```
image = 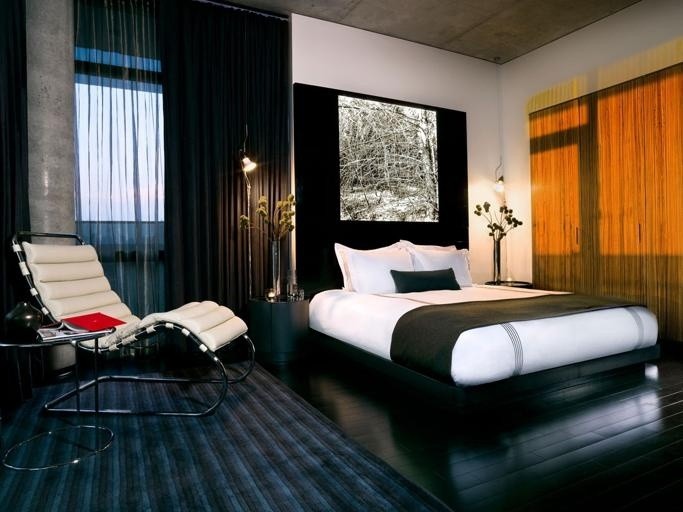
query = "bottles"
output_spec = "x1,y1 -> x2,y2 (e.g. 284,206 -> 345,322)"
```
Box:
5,301 -> 45,345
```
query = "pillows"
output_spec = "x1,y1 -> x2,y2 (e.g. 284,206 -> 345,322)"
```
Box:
335,239 -> 474,293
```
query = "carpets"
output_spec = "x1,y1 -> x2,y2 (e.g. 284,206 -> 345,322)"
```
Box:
0,360 -> 453,512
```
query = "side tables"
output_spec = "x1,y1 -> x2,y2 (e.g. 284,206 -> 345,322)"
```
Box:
0,324 -> 115,471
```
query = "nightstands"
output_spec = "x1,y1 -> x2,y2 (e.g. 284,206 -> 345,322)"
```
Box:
485,280 -> 532,290
254,299 -> 309,363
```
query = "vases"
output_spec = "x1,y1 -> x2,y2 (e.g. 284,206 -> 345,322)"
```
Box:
494,240 -> 501,284
272,240 -> 281,302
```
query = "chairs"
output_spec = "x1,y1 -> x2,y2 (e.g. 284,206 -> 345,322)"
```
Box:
13,230 -> 256,417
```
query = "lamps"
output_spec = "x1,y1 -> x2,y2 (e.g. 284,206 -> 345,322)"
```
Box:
241,155 -> 258,300
496,176 -> 515,282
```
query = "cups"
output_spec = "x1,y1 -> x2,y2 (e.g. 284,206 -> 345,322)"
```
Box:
264,289 -> 277,303
286,283 -> 297,297
294,289 -> 305,301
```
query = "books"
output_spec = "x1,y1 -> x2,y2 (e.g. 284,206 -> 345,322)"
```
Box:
41,312 -> 127,333
35,328 -> 115,342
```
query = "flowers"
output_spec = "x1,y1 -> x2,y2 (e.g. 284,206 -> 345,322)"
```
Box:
239,194 -> 296,281
474,202 -> 523,278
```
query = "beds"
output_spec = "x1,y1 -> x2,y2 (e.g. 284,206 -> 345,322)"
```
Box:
309,284 -> 662,388
